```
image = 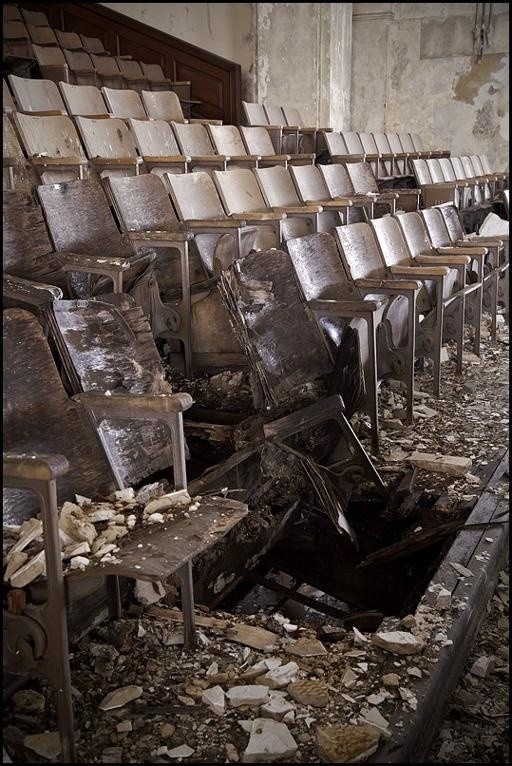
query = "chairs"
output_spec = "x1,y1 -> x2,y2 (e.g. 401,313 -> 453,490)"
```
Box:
1,111 -> 510,500
2,6 -> 24,23
242,101 -> 305,128
21,8 -> 50,26
2,74 -> 223,124
2,23 -> 131,61
1,308 -> 250,765
3,43 -> 192,116
